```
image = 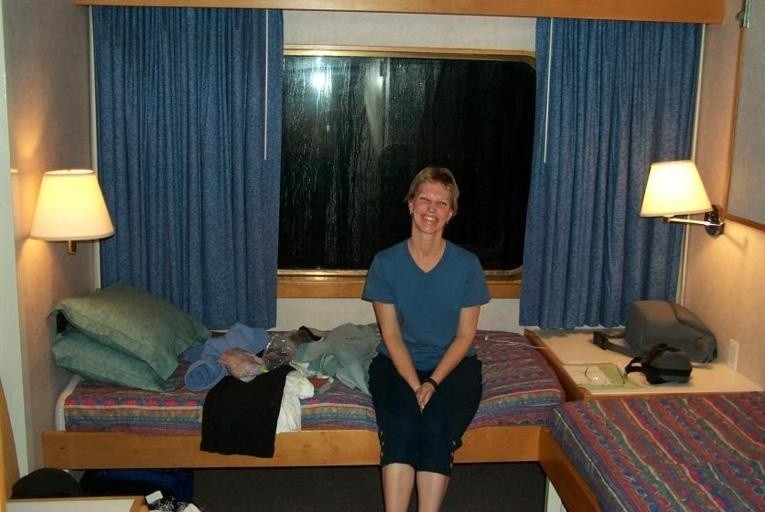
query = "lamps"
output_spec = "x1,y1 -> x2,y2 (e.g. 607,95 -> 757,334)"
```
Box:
20,167 -> 118,258
637,156 -> 727,238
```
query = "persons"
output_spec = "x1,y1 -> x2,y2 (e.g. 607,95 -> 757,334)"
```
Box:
289,95 -> 365,268
362,166 -> 491,511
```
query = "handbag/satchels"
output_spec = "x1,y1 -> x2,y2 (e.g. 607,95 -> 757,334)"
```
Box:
624,344 -> 692,385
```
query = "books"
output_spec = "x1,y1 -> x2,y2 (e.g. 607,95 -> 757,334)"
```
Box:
562,361 -> 643,395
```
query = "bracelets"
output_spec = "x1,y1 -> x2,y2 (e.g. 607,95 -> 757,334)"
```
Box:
423,377 -> 439,391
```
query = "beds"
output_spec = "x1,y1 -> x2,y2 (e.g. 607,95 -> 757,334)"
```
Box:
42,327 -> 566,475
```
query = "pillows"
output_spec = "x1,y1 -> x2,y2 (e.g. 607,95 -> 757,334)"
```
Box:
48,278 -> 214,398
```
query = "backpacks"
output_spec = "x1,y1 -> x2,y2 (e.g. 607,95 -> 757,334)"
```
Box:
592,300 -> 717,363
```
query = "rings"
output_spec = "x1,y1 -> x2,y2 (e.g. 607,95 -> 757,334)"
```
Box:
419,400 -> 424,405
